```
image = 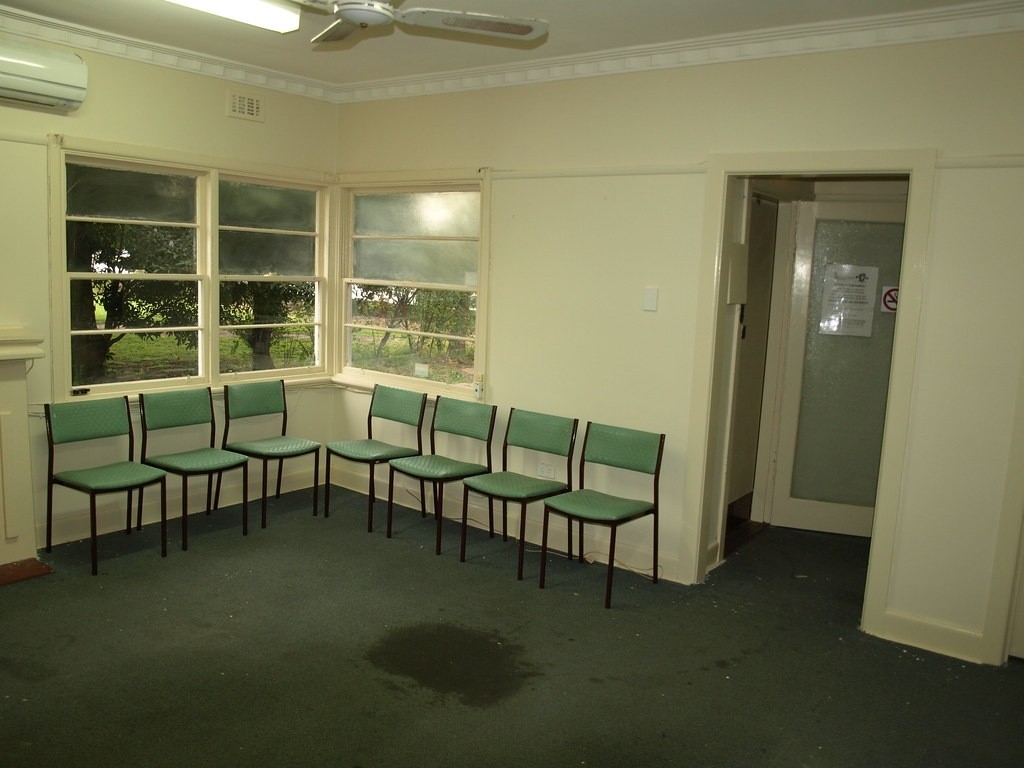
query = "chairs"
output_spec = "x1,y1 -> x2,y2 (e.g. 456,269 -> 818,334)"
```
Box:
213,378 -> 322,530
537,419 -> 668,609
386,394 -> 498,556
459,406 -> 581,581
324,382 -> 428,534
136,385 -> 251,553
40,392 -> 169,576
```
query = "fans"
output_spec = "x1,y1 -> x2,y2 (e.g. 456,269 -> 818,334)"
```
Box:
264,0 -> 552,47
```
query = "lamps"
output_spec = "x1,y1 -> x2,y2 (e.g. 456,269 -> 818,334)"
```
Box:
163,0 -> 302,35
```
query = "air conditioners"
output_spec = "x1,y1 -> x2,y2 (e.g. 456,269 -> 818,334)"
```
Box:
0,42 -> 90,116
